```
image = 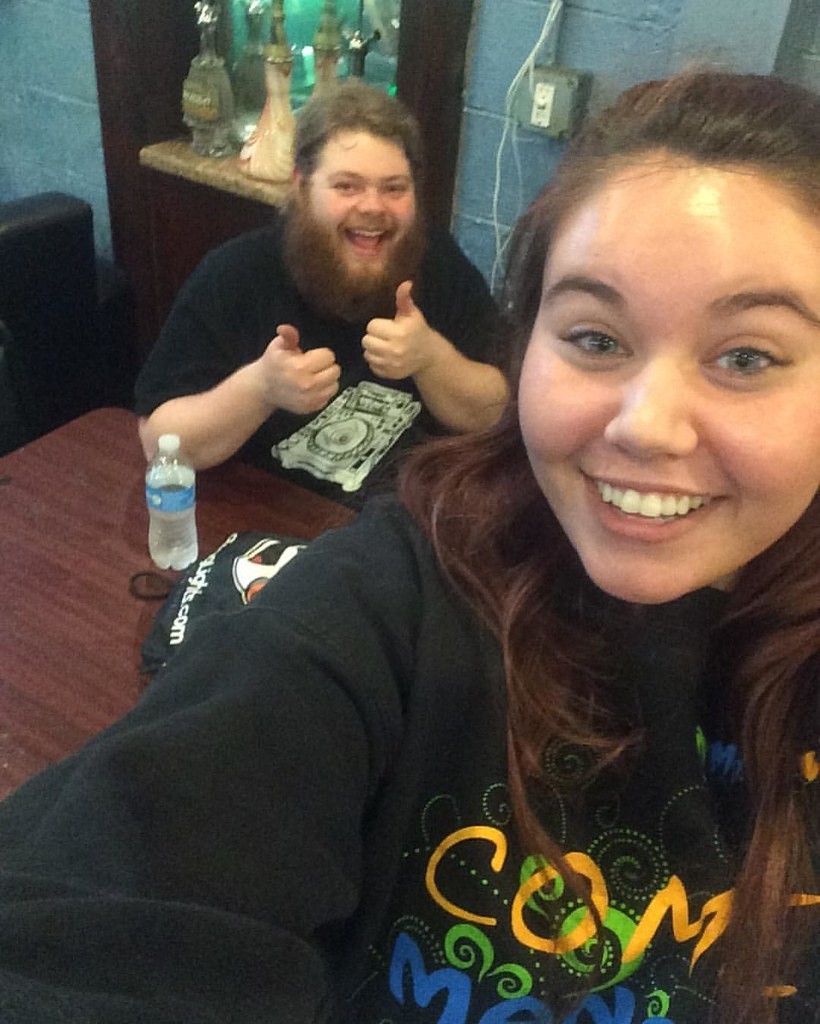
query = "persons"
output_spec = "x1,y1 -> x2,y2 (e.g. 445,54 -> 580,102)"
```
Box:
0,71 -> 820,1024
134,85 -> 510,511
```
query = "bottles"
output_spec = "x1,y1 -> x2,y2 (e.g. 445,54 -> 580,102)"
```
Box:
143,433 -> 199,571
227,0 -> 269,146
239,1 -> 298,180
311,0 -> 344,97
179,0 -> 235,154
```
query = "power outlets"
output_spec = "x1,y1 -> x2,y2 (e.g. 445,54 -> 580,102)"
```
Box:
515,64 -> 591,139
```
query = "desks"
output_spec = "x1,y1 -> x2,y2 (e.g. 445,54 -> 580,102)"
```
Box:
0,405 -> 361,811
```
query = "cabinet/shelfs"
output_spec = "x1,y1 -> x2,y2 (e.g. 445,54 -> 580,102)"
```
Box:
131,178 -> 290,336
